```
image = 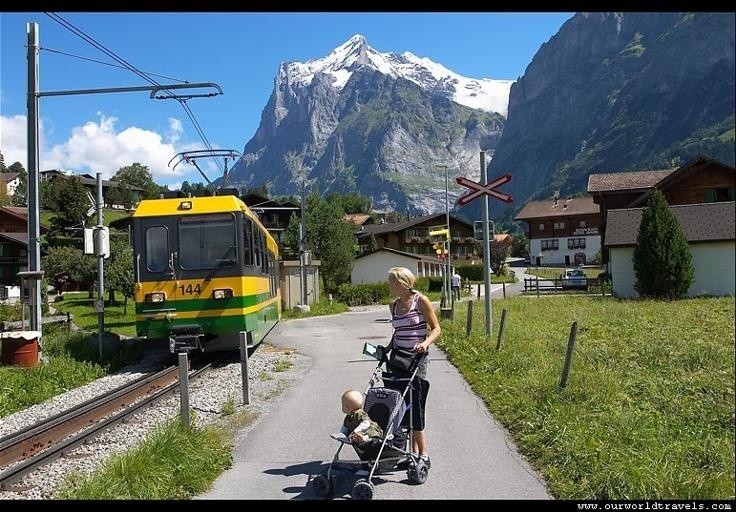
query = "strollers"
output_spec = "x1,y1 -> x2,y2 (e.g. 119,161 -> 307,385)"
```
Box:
311,344 -> 435,503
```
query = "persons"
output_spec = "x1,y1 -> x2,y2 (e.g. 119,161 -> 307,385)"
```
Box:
328,293 -> 333,306
374,266 -> 442,469
451,269 -> 462,302
340,388 -> 386,460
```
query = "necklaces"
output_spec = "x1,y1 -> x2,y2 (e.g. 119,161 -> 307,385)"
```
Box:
402,307 -> 406,311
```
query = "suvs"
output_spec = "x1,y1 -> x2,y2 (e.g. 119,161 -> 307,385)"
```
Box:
559,267 -> 589,290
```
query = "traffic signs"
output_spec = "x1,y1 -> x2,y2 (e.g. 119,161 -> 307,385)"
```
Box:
428,224 -> 448,235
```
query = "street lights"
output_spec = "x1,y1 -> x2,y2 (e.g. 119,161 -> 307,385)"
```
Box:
433,161 -> 452,321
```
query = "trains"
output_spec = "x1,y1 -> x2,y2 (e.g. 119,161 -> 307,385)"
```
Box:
127,196 -> 284,354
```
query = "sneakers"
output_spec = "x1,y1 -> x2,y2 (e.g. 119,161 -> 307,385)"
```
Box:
399,455 -> 431,470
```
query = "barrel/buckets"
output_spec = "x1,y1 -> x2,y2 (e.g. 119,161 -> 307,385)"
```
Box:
2,339 -> 38,368
441,308 -> 451,320
